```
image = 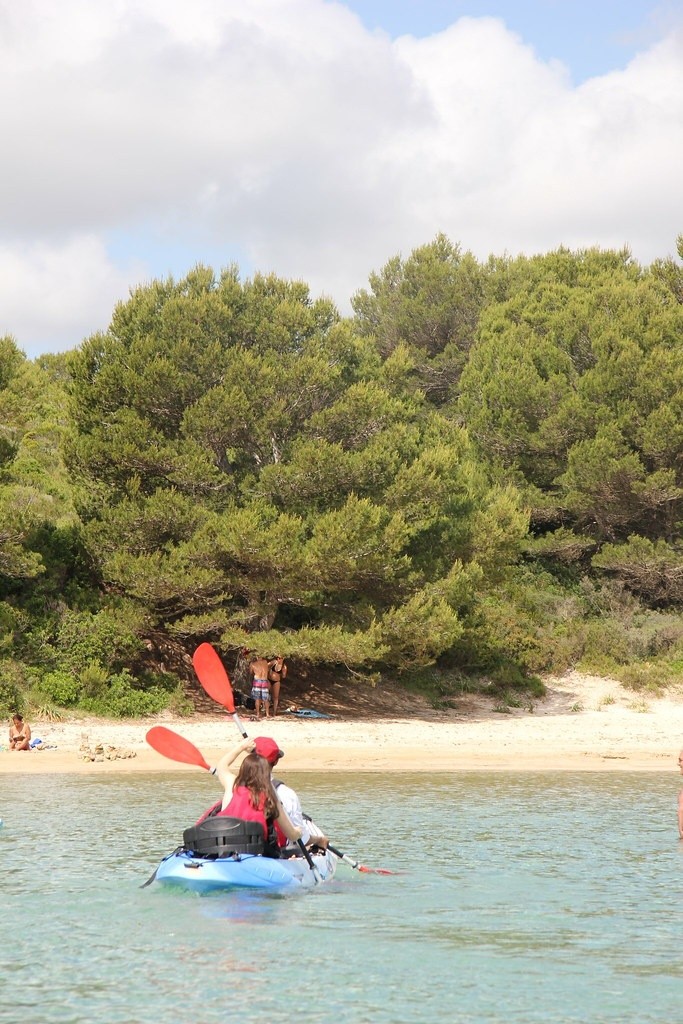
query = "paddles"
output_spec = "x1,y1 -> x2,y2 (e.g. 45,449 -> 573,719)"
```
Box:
145,727 -> 398,875
192,642 -> 327,888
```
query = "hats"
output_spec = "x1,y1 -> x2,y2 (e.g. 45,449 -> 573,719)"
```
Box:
254,737 -> 284,764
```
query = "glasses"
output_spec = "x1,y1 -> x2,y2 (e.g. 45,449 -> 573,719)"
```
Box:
279,658 -> 283,660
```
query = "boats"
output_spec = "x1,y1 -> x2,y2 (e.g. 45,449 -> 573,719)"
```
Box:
155,849 -> 337,896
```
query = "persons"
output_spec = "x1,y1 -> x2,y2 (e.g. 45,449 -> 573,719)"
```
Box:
249,655 -> 270,720
216,737 -> 306,843
267,655 -> 287,717
8,715 -> 31,752
677,746 -> 683,839
253,737 -> 330,850
